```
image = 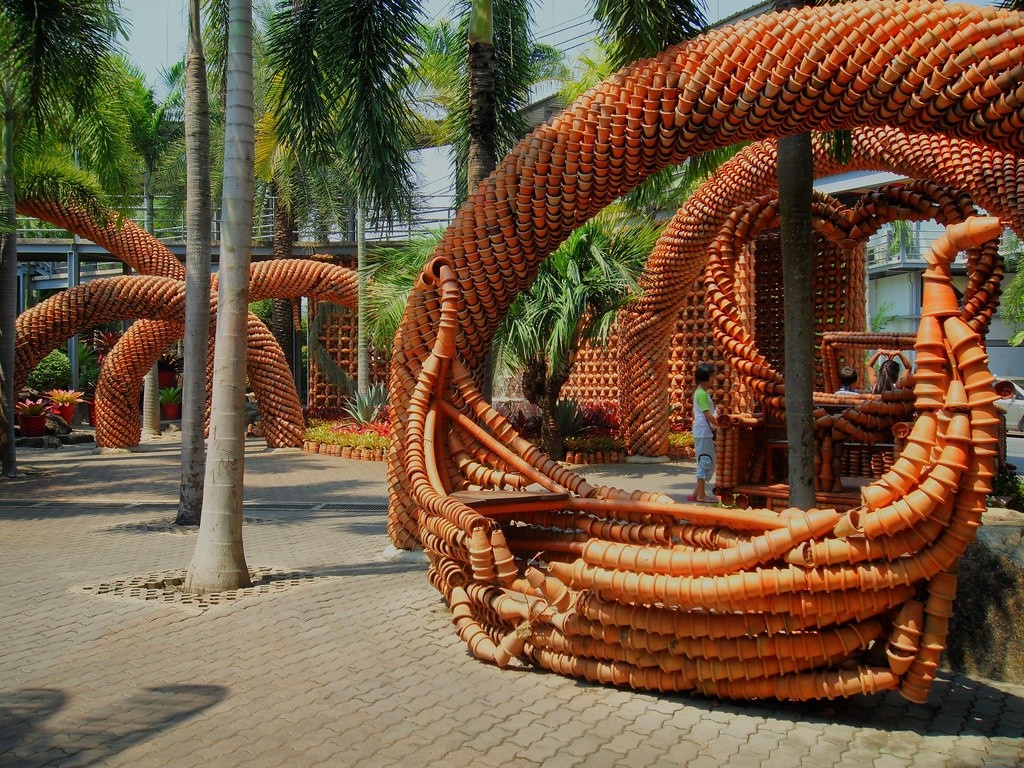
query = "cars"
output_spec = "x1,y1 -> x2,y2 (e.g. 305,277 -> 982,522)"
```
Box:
993,379 -> 1024,433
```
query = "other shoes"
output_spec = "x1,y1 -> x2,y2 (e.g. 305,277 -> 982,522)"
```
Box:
700,496 -> 720,503
688,494 -> 697,501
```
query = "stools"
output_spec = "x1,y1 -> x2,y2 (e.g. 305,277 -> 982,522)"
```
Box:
445,486 -> 570,517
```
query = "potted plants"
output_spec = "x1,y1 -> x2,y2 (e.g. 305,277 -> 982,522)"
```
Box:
157,355 -> 184,386
85,382 -> 96,427
159,387 -> 182,420
15,399 -> 53,437
39,389 -> 94,426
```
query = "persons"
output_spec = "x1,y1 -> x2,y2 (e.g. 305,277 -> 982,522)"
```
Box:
867,350 -> 912,394
834,366 -> 859,394
687,364 -> 718,503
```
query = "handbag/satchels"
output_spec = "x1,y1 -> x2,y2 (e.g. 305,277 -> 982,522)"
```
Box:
711,428 -> 719,441
